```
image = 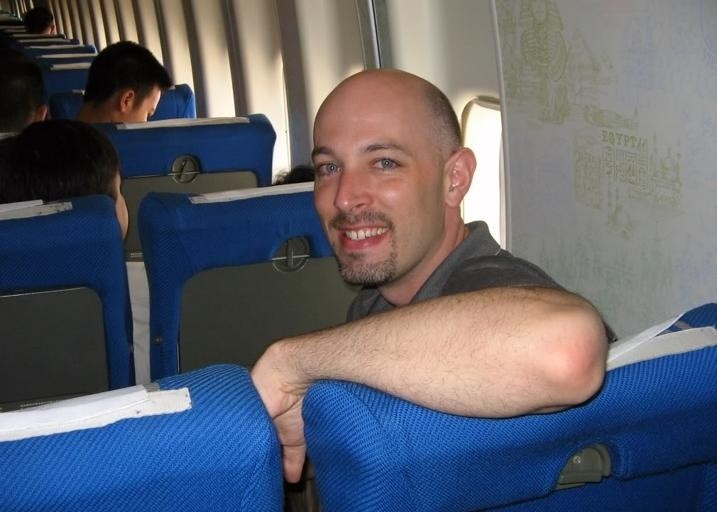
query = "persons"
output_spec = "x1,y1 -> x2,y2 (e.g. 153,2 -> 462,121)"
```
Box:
251,69 -> 619,485
73,41 -> 176,123
269,164 -> 315,186
0,63 -> 48,130
24,6 -> 55,34
1,120 -> 129,254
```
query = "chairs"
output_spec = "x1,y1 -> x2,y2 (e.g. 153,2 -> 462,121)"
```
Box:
1,12 -> 717,510
